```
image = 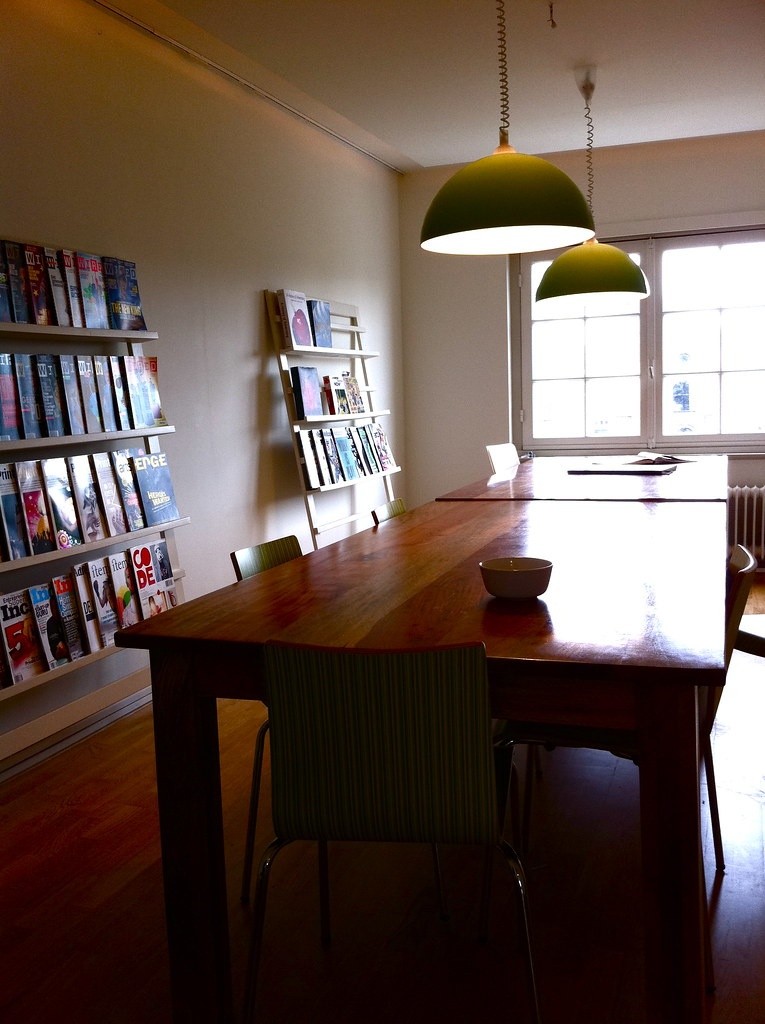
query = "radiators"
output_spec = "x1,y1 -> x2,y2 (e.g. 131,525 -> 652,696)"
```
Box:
728,484 -> 765,560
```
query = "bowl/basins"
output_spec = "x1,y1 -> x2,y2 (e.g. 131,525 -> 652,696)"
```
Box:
479,557 -> 553,601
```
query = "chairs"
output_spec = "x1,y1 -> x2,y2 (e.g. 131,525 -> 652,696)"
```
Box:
478,544 -> 758,914
236,640 -> 540,1024
229,536 -> 522,920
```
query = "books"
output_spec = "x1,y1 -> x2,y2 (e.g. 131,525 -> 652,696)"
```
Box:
0,239 -> 149,331
0,446 -> 182,561
274,287 -> 333,348
0,352 -> 169,442
288,364 -> 323,420
294,422 -> 396,491
0,538 -> 180,685
322,373 -> 365,415
566,451 -> 698,475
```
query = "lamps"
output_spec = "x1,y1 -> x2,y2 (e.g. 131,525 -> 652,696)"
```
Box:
419,0 -> 597,256
536,63 -> 652,303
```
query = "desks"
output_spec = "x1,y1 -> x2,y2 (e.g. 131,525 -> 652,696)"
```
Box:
113,502 -> 726,1024
434,454 -> 729,502
